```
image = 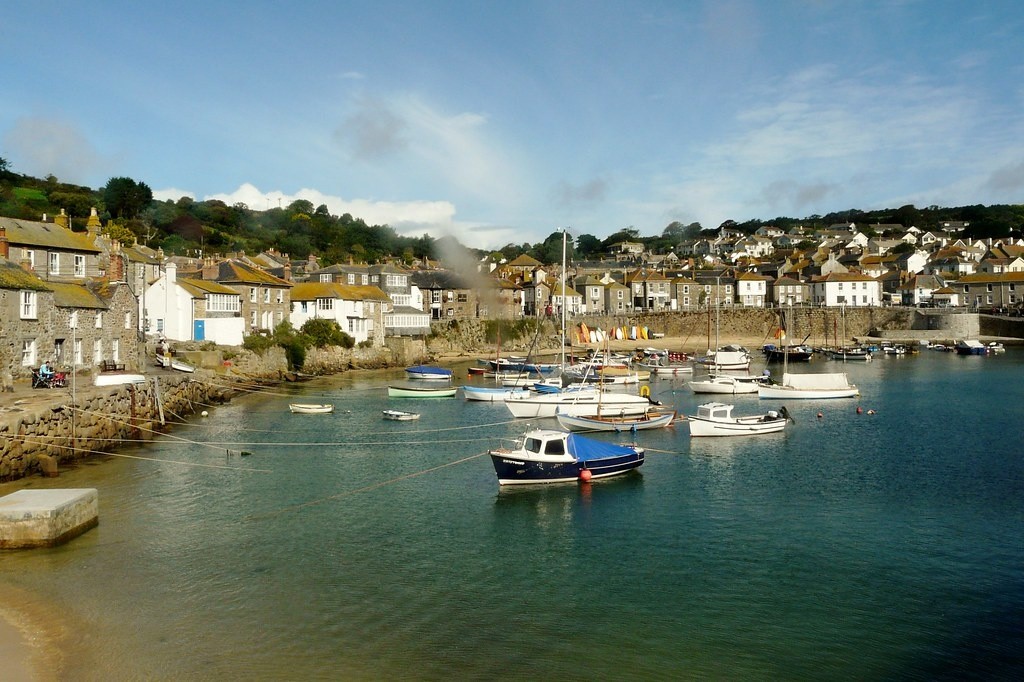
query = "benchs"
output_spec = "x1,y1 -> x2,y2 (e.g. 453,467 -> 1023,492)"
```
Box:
104,360 -> 125,371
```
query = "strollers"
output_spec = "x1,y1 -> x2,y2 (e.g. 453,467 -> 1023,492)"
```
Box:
29,366 -> 51,388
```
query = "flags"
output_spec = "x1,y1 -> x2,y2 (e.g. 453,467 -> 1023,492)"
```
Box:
774,323 -> 787,340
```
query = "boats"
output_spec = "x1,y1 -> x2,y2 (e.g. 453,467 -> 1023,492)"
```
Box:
289,402 -> 335,413
462,339 -> 985,438
406,365 -> 454,379
482,428 -> 648,491
386,383 -> 458,398
155,354 -> 195,373
381,408 -> 421,422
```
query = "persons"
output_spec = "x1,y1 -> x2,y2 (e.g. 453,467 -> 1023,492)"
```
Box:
158,336 -> 170,369
35,370 -> 40,378
39,361 -> 55,378
